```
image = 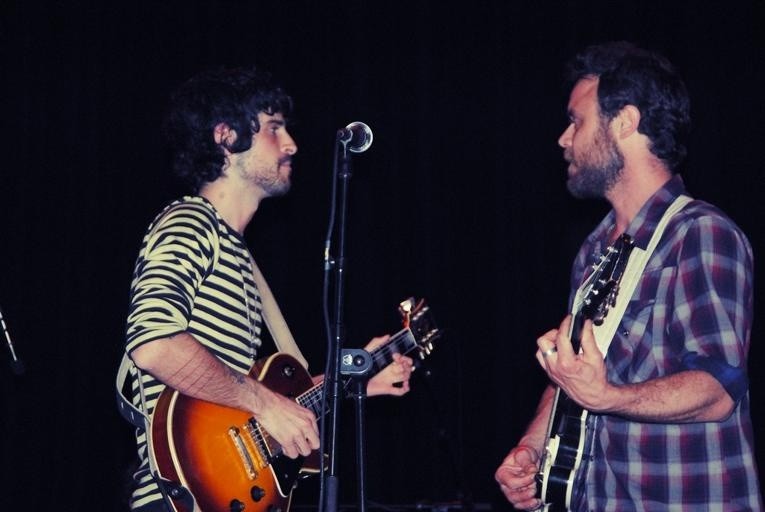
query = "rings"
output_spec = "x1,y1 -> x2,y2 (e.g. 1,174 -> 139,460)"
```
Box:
541,346 -> 557,358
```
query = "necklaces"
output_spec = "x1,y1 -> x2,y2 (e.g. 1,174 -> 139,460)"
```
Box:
222,221 -> 260,369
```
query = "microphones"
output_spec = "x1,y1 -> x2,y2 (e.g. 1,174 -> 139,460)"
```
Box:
336,119 -> 375,156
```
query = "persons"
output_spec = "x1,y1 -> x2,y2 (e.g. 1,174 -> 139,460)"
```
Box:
493,38 -> 763,511
123,62 -> 415,512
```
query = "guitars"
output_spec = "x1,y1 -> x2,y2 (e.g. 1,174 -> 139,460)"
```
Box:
150,295 -> 441,512
536,232 -> 638,511
